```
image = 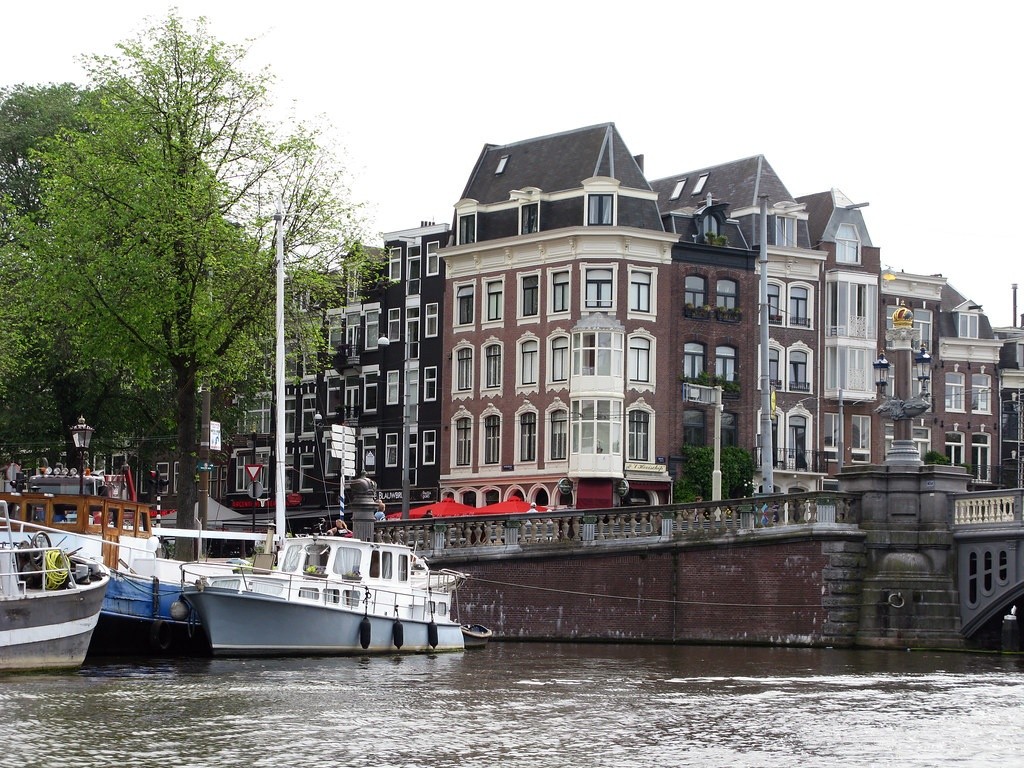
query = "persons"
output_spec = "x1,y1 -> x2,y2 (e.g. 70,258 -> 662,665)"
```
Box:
420,509 -> 433,530
526,502 -> 538,513
694,496 -> 703,521
374,503 -> 386,522
374,499 -> 383,515
327,519 -> 353,538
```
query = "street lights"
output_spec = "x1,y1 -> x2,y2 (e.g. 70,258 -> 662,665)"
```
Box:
69,415 -> 95,494
724,190 -> 774,495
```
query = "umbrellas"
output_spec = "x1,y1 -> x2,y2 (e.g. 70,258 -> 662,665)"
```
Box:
474,495 -> 549,516
393,497 -> 475,517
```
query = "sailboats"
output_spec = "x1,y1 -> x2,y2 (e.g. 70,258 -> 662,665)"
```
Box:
178,192 -> 474,659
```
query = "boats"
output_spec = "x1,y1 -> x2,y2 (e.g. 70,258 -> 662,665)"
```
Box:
459,623 -> 493,649
0,517 -> 305,657
0,499 -> 111,674
